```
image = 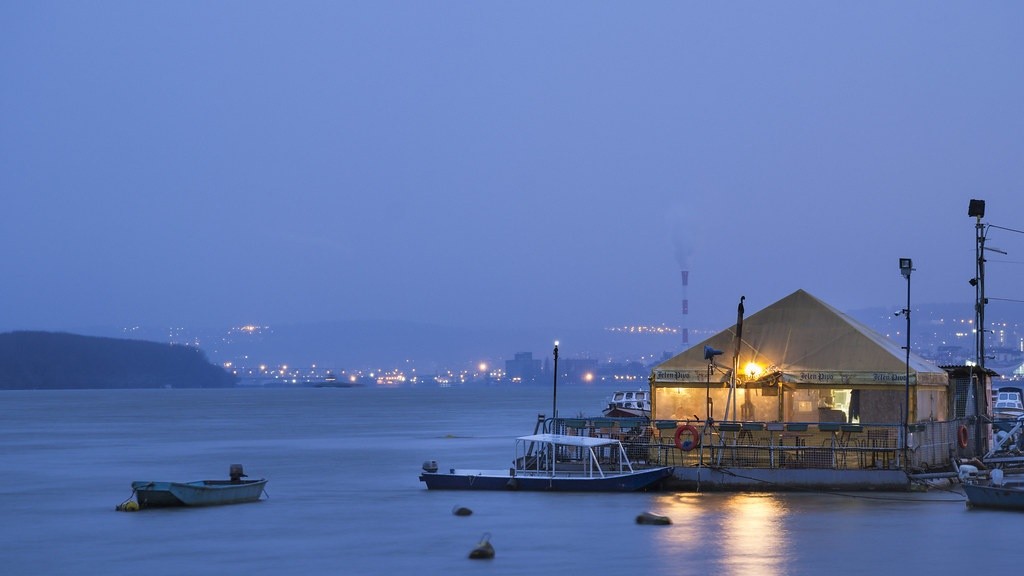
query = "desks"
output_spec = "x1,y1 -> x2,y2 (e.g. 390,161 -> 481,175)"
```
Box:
784,434 -> 814,462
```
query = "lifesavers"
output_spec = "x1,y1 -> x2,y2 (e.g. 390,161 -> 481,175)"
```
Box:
617,402 -> 644,408
958,425 -> 970,448
674,425 -> 699,451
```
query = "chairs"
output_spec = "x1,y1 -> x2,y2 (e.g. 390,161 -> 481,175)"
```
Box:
860,429 -> 890,470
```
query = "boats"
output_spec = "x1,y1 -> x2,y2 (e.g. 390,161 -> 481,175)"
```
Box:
602,386 -> 652,419
419,433 -> 676,493
116,463 -> 268,513
957,386 -> 1024,512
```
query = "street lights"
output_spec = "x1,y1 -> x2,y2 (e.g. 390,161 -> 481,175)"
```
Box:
552,340 -> 560,417
894,257 -> 917,470
968,198 -> 987,367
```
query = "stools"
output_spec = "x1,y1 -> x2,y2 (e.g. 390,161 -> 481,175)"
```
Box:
843,438 -> 861,469
661,436 -> 684,467
821,437 -> 844,470
